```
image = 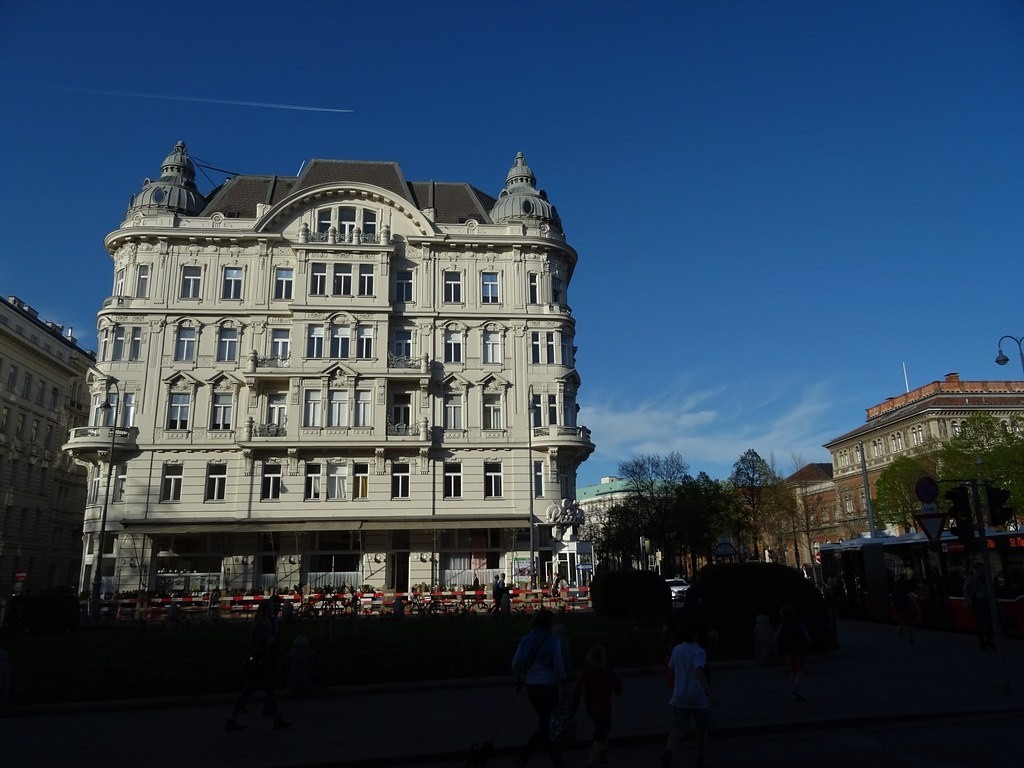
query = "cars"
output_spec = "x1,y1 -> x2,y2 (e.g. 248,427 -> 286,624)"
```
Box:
802,564 -> 821,583
665,578 -> 691,600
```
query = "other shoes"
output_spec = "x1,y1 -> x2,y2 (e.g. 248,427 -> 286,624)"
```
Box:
792,684 -> 808,702
985,638 -> 997,651
273,718 -> 293,729
514,756 -> 526,768
562,758 -> 568,767
696,758 -> 704,768
793,695 -> 800,703
661,751 -> 672,768
598,760 -> 615,768
909,639 -> 918,646
898,631 -> 904,639
224,718 -> 247,731
586,762 -> 597,768
979,643 -> 989,651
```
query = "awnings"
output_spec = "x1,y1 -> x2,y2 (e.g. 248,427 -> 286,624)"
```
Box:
121,515 -> 533,548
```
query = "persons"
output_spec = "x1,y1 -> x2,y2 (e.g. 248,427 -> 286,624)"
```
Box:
512,610 -> 624,768
225,596 -> 294,731
109,594 -> 120,622
552,574 -> 568,607
964,562 -> 997,651
895,568 -> 921,647
775,606 -> 813,703
283,591 -> 293,616
493,573 -> 509,614
662,589 -> 720,768
808,570 -> 863,617
474,573 -> 479,587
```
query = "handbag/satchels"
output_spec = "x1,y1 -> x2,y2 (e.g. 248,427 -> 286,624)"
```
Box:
913,608 -> 922,623
548,680 -> 577,744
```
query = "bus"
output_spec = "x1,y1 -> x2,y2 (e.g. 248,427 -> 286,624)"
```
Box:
820,526 -> 1024,638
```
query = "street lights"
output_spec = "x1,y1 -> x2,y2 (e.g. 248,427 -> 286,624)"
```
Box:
995,335 -> 1024,374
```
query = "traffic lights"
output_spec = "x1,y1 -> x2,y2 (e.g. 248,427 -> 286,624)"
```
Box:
985,486 -> 1013,526
945,485 -> 975,540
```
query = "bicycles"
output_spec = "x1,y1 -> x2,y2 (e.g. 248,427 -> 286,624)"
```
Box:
468,601 -> 502,617
297,596 -> 339,616
403,595 -> 447,615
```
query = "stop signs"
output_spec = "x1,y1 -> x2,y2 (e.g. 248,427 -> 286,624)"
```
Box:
14,567 -> 28,581
816,552 -> 821,562
